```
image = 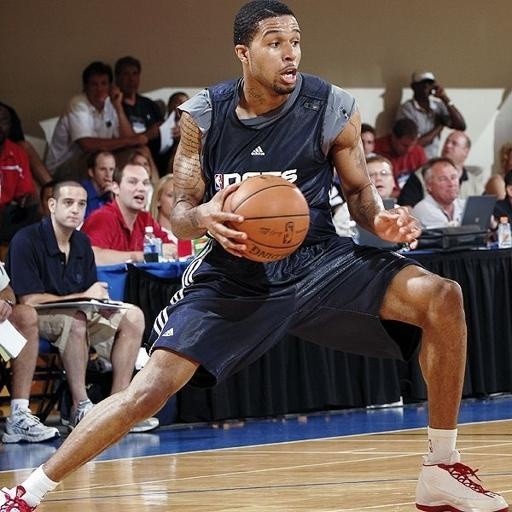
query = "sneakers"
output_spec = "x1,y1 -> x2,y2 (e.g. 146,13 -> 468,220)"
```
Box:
68,399 -> 94,427
129,417 -> 160,433
415,449 -> 510,511
0,485 -> 41,512
1,408 -> 61,444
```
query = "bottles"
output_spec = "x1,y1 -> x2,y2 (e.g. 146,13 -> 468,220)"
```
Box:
497,217 -> 512,249
143,226 -> 158,263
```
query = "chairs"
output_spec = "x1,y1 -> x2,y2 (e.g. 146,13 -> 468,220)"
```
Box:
0,322 -> 101,426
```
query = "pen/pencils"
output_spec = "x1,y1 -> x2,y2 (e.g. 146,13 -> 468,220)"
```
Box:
88,283 -> 112,289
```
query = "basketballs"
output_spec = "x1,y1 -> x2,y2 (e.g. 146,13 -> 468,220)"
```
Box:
222,175 -> 310,263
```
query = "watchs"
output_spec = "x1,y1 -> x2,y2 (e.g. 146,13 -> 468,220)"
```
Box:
5,299 -> 14,306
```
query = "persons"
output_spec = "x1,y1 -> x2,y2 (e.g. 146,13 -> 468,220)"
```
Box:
115,57 -> 164,181
325,70 -> 512,249
39,149 -> 192,266
1,0 -> 509,511
6,181 -> 160,436
1,103 -> 54,230
1,260 -> 60,444
168,92 -> 191,116
45,61 -> 165,181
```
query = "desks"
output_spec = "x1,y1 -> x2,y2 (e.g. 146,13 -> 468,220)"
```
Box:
97,235 -> 512,429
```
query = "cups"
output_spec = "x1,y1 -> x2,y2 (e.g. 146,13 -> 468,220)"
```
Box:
164,244 -> 177,262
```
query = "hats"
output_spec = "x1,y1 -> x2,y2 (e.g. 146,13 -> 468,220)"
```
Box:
412,69 -> 435,83
